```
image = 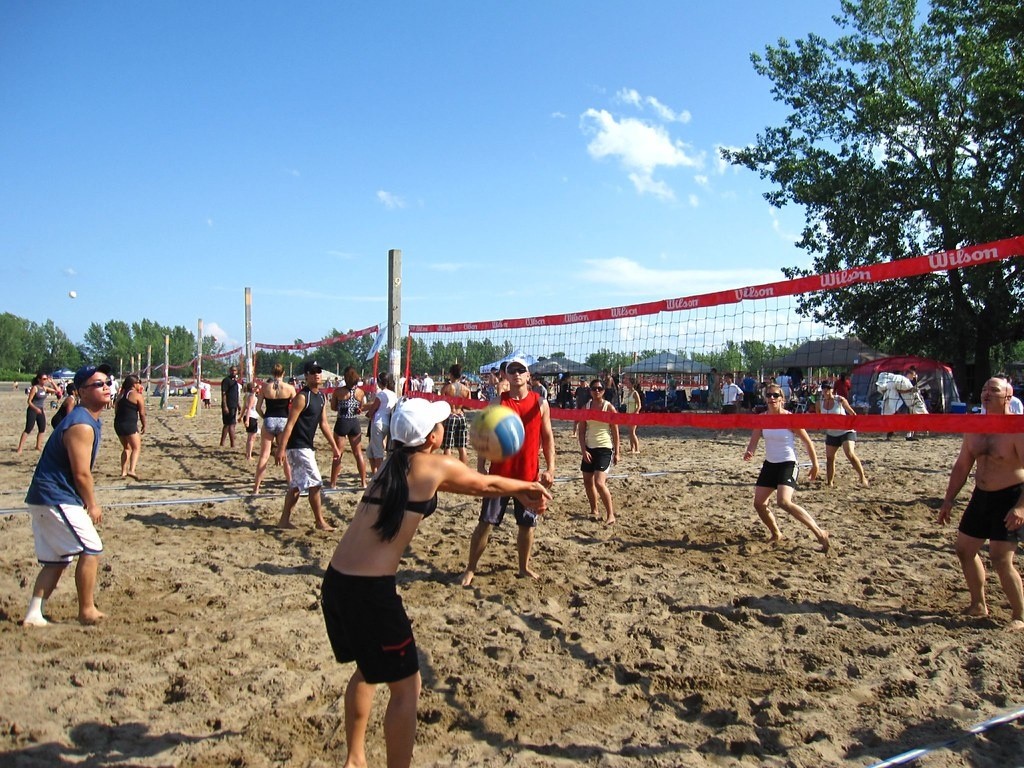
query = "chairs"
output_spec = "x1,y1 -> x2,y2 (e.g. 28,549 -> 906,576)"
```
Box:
603,387 -> 709,411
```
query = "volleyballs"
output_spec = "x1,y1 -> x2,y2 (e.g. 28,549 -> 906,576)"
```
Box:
469,407 -> 527,460
69,291 -> 76,297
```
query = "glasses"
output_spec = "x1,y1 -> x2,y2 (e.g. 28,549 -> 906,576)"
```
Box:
590,386 -> 605,392
39,377 -> 49,381
821,386 -> 834,391
506,366 -> 526,374
765,393 -> 781,398
306,368 -> 321,374
85,381 -> 112,388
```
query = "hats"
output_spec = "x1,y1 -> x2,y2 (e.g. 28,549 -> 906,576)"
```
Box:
73,363 -> 112,389
579,376 -> 588,382
821,380 -> 835,388
390,398 -> 451,447
303,361 -> 324,370
505,357 -> 528,372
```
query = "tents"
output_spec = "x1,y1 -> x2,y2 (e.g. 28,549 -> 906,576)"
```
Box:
480,346 -> 539,373
527,356 -> 600,375
851,354 -> 961,414
293,368 -> 342,381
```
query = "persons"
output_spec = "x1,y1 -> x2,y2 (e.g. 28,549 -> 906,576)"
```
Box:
458,360 -> 555,587
935,362 -> 1024,631
17,362 -> 146,628
885,366 -> 919,441
666,368 -> 758,438
320,396 -> 552,768
554,371 -> 641,525
200,362 -> 296,495
740,367 -> 869,551
274,360 -> 399,531
402,364 -> 471,464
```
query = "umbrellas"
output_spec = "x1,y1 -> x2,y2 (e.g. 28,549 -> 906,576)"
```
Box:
623,349 -> 712,408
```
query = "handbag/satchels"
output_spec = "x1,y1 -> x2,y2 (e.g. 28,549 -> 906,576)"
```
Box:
897,403 -> 911,415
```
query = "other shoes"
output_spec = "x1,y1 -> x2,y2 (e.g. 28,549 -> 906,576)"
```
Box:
907,436 -> 921,442
